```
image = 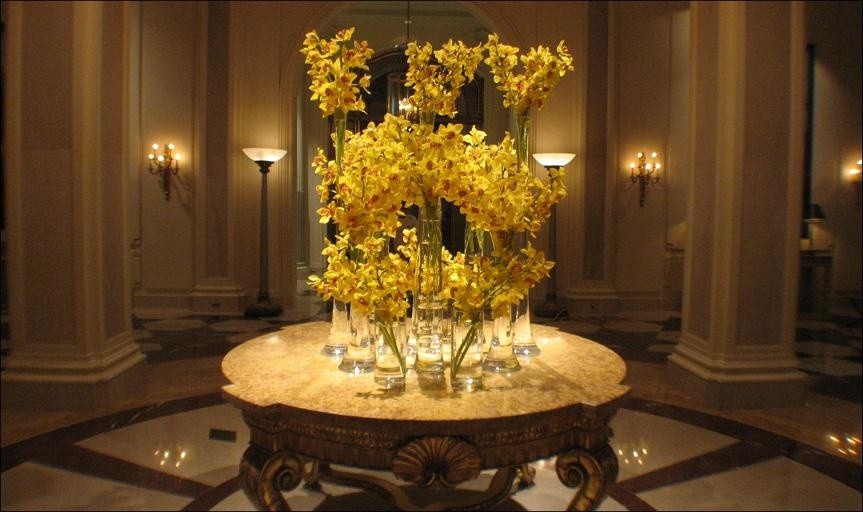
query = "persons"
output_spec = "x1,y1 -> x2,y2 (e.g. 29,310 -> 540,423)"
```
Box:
393,201 -> 417,254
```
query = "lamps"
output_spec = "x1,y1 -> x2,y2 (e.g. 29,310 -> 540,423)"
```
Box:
148,142 -> 180,202
630,150 -> 661,208
387,0 -> 435,127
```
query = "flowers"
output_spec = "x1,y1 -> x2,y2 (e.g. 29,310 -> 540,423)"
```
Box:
293,23 -> 575,376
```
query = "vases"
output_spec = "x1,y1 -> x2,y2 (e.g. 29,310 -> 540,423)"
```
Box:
371,314 -> 411,391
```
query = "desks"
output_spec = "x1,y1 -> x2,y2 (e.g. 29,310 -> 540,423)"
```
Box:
220,318 -> 632,512
